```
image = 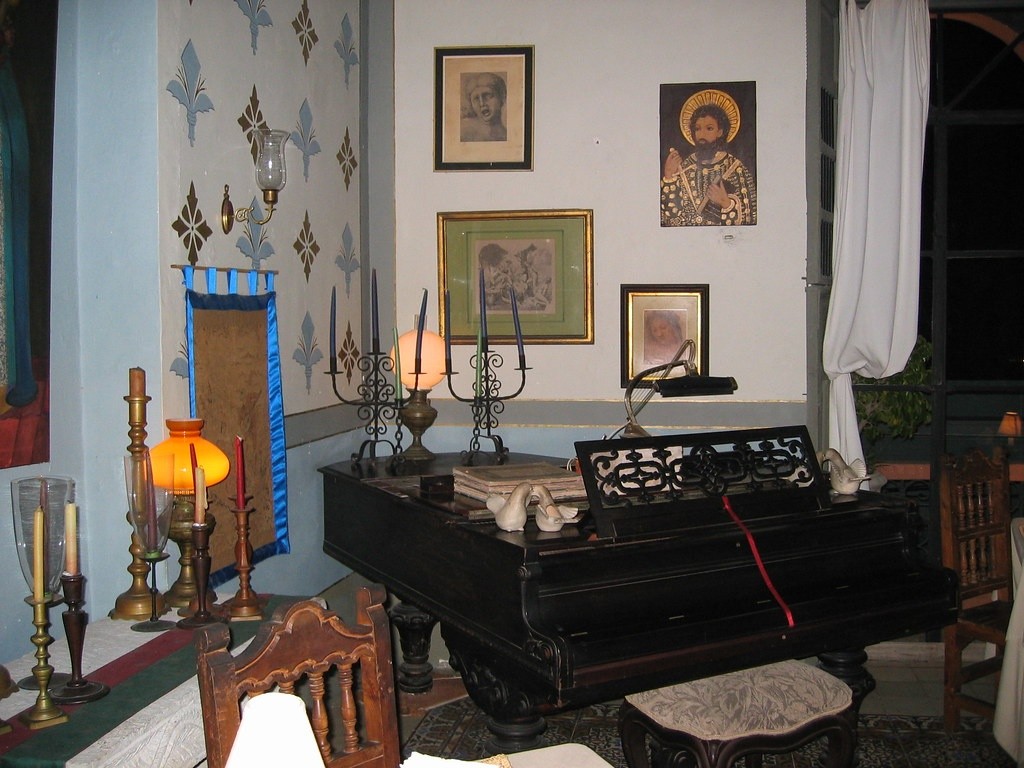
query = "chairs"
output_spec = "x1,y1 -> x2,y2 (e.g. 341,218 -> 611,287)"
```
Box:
193,583 -> 400,767
937,448 -> 1013,726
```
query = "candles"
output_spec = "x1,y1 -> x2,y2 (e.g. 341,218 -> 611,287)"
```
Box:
372,269 -> 379,339
34,480 -> 47,599
146,453 -> 157,550
478,269 -> 487,338
235,436 -> 245,510
476,329 -> 482,397
415,288 -> 428,359
444,291 -> 451,359
190,443 -> 208,524
129,366 -> 145,397
65,500 -> 77,574
330,286 -> 336,358
510,288 -> 524,356
392,328 -> 402,399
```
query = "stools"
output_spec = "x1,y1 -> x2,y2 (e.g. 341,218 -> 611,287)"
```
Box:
618,660 -> 857,768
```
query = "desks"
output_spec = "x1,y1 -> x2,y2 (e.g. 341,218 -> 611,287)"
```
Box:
0,591 -> 327,768
508,743 -> 615,768
873,457 -> 1024,481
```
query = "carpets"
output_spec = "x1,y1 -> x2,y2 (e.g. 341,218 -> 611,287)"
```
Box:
399,695 -> 1018,768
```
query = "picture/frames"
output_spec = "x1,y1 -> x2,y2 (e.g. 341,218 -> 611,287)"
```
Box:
620,284 -> 710,388
434,44 -> 535,171
437,209 -> 594,345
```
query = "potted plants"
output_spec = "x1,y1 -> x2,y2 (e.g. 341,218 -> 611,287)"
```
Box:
850,335 -> 932,457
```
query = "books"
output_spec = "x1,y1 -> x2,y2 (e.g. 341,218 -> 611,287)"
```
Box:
453,462 -> 588,504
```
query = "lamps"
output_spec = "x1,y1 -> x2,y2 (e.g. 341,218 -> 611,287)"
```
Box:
390,329 -> 445,461
144,417 -> 230,607
997,412 -> 1022,446
222,130 -> 291,234
224,692 -> 325,768
619,339 -> 738,439
